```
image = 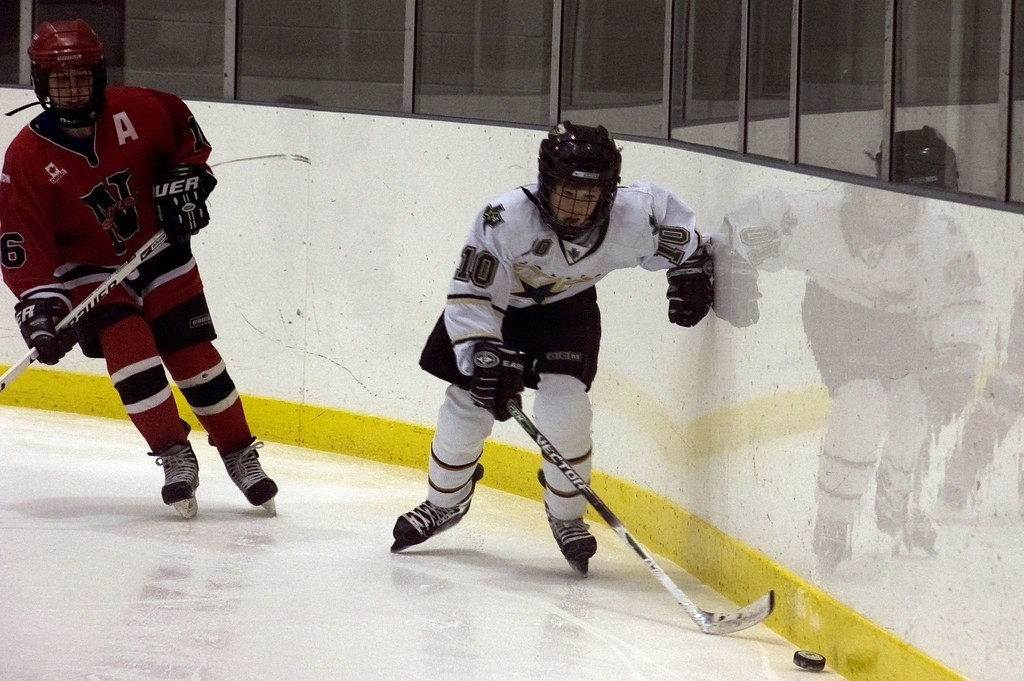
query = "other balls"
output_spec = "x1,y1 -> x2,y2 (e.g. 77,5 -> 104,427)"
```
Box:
794,649 -> 825,671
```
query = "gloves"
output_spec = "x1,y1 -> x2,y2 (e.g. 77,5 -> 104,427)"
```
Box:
923,366 -> 975,425
666,254 -> 714,327
15,298 -> 81,365
468,340 -> 527,423
152,163 -> 210,247
708,254 -> 762,328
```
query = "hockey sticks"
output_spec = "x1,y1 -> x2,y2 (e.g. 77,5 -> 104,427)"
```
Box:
508,401 -> 777,636
0,230 -> 171,390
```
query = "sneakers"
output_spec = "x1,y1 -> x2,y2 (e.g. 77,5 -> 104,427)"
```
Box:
938,465 -> 979,514
147,418 -> 199,520
811,517 -> 849,584
537,468 -> 597,578
390,463 -> 484,553
208,433 -> 278,515
876,508 -> 937,558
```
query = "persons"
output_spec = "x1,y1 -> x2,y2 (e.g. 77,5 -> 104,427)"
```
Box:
394,122 -> 714,560
0,18 -> 277,520
714,125 -> 984,565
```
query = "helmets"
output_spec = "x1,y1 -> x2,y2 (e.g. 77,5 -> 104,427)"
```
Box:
874,124 -> 960,194
275,94 -> 316,106
26,18 -> 107,110
536,120 -> 622,241
